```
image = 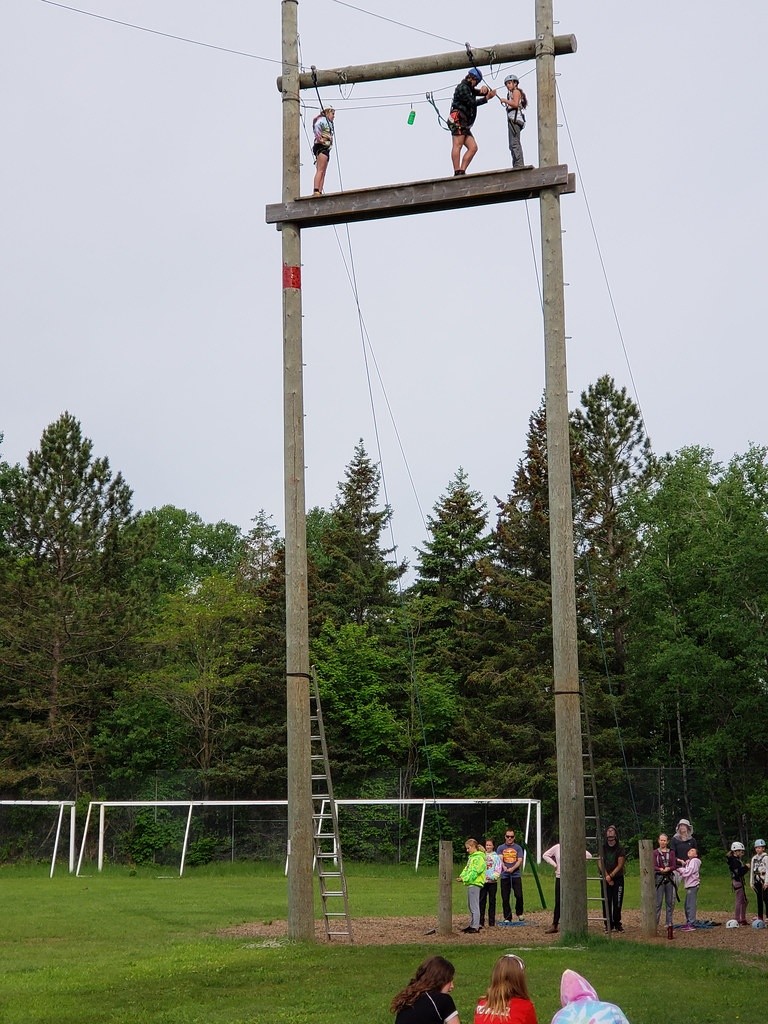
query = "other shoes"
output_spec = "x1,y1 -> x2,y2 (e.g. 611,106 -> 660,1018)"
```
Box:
681,923 -> 697,932
504,919 -> 510,923
615,926 -> 624,933
604,925 -> 614,933
519,915 -> 524,921
667,926 -> 673,940
461,926 -> 480,933
544,923 -> 558,934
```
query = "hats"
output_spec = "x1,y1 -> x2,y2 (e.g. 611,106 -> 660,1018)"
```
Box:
605,825 -> 619,839
676,818 -> 693,836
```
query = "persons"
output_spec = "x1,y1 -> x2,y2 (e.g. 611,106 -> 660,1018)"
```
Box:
551,970 -> 629,1024
676,848 -> 701,932
500,75 -> 526,167
448,69 -> 497,175
497,830 -> 524,922
456,839 -> 486,934
749,839 -> 768,921
670,819 -> 697,901
479,840 -> 502,929
313,104 -> 334,196
727,842 -> 752,926
475,954 -> 538,1024
654,833 -> 676,939
543,844 -> 592,933
597,825 -> 625,933
391,957 -> 461,1024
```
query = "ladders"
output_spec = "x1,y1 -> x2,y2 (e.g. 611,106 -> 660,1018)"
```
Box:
303,663 -> 355,944
579,678 -> 612,940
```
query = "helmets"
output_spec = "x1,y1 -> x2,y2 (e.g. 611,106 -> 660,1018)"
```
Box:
752,920 -> 765,929
754,839 -> 766,847
468,68 -> 483,83
731,841 -> 745,851
320,104 -> 335,115
504,74 -> 520,84
725,919 -> 739,929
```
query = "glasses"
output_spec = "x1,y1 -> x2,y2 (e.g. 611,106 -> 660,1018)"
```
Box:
506,953 -> 524,969
506,835 -> 514,839
